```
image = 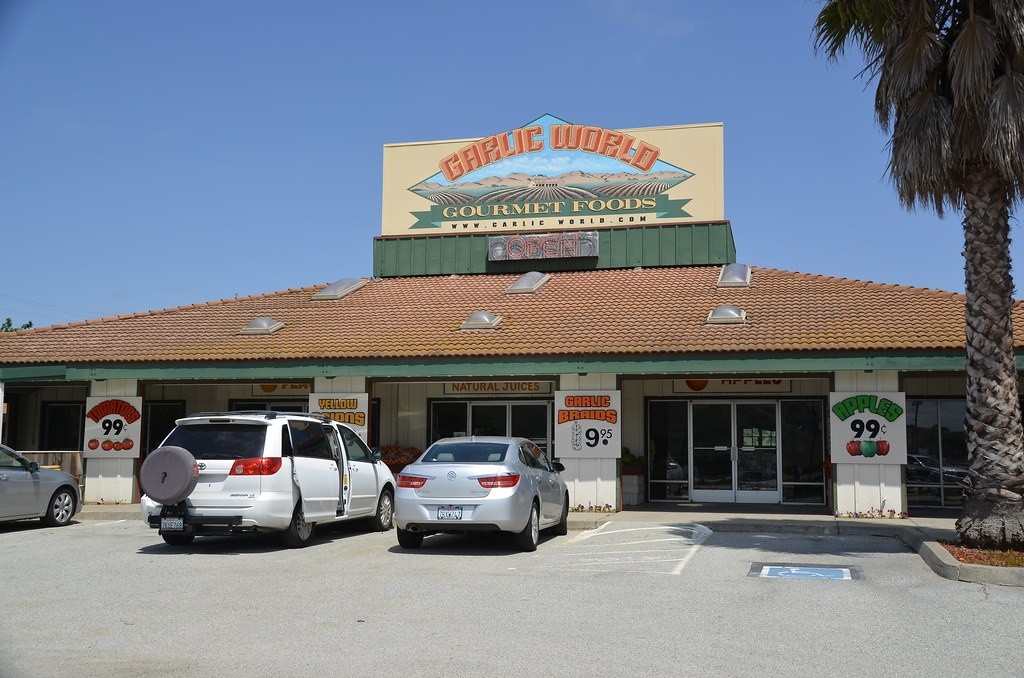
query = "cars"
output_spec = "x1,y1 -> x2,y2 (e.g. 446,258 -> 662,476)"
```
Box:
394,435 -> 570,552
905,454 -> 970,496
0,443 -> 83,527
666,453 -> 685,497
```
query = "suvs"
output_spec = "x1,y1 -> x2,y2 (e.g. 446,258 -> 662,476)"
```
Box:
139,410 -> 398,547
